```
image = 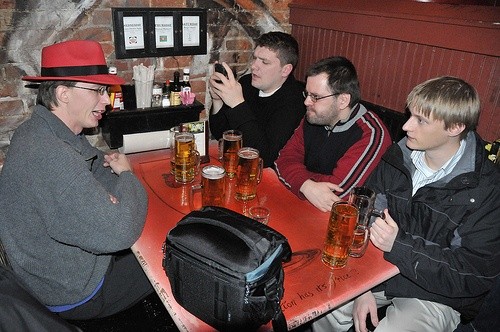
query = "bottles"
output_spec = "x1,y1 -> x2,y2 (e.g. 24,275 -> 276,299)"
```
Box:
152,95 -> 161,107
162,94 -> 170,107
163,80 -> 171,94
108,67 -> 123,110
180,68 -> 191,93
170,71 -> 181,106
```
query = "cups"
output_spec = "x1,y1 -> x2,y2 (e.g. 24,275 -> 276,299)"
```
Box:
348,186 -> 386,247
170,126 -> 200,184
249,206 -> 270,225
188,164 -> 226,211
320,200 -> 372,270
218,130 -> 243,179
234,147 -> 263,203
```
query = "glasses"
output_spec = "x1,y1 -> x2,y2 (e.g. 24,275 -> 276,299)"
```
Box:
69,85 -> 109,96
302,90 -> 339,103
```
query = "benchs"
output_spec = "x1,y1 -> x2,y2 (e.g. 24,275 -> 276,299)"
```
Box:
0,257 -> 181,332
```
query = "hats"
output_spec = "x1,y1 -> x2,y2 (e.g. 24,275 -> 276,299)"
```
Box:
22,41 -> 126,85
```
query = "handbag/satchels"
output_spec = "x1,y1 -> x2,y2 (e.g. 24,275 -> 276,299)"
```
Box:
162,206 -> 293,332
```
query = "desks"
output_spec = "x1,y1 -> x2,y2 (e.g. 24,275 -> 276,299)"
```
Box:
129,139 -> 400,332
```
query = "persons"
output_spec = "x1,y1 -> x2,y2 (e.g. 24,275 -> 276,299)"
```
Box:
0,39 -> 155,321
208,31 -> 307,168
312,76 -> 500,332
274,56 -> 392,213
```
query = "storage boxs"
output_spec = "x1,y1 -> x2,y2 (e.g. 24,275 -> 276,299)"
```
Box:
101,98 -> 204,149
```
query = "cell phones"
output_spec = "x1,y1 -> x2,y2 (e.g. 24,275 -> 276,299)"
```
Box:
214,63 -> 226,83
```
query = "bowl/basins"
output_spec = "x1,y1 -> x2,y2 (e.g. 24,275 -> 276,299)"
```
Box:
180,93 -> 195,105
